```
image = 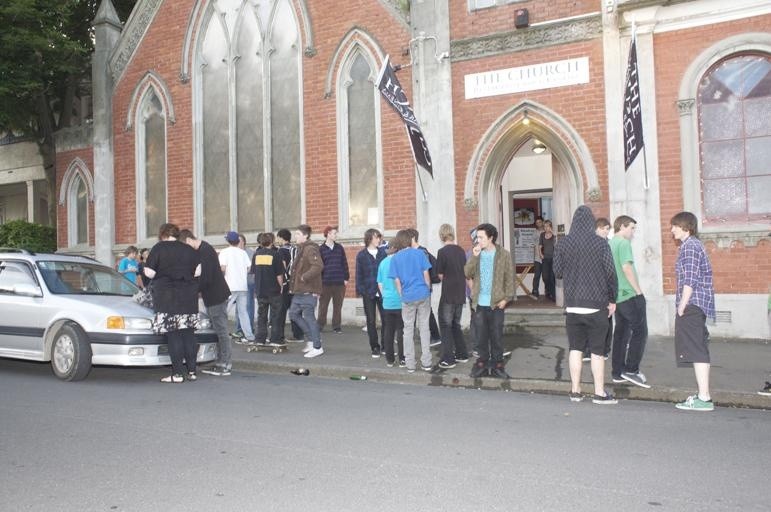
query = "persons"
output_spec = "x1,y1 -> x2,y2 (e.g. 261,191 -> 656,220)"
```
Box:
219,225 -> 350,358
118,245 -> 151,290
529,216 -> 555,302
144,223 -> 202,383
354,223 -> 515,380
758,284 -> 771,401
553,207 -> 651,403
670,211 -> 715,411
177,229 -> 232,376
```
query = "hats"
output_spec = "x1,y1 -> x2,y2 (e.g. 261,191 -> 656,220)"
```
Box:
223,231 -> 241,242
323,226 -> 338,236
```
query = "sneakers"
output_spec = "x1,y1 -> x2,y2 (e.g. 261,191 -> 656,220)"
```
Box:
371,340 -> 510,378
333,326 -> 342,334
160,367 -> 232,382
676,396 -> 715,412
568,390 -> 583,403
229,322 -> 325,357
756,382 -> 771,397
582,352 -> 651,388
591,393 -> 619,405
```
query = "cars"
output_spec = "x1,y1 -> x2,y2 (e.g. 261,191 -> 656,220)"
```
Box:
0,247 -> 219,382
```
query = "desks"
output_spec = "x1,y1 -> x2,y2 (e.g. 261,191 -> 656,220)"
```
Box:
514,262 -> 535,295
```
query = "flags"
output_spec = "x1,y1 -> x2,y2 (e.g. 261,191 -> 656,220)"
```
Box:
621,14 -> 647,174
371,52 -> 436,181
